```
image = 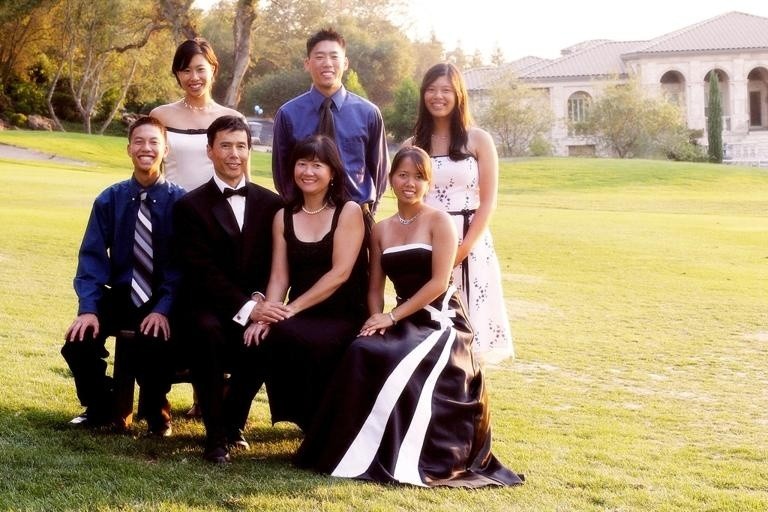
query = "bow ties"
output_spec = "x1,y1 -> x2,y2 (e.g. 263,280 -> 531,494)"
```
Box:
223,186 -> 249,198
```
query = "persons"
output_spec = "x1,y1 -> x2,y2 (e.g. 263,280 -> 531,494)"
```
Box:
167,116 -> 286,463
401,61 -> 518,366
60,117 -> 189,437
272,29 -> 389,220
244,136 -> 366,474
291,145 -> 524,488
148,38 -> 251,193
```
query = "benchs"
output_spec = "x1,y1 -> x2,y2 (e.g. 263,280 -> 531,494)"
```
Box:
109,330 -> 137,427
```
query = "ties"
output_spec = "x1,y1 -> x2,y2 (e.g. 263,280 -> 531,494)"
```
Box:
316,97 -> 336,143
131,190 -> 155,308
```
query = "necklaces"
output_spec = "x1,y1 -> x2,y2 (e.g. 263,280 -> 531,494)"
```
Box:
397,211 -> 420,224
431,134 -> 448,142
301,202 -> 329,214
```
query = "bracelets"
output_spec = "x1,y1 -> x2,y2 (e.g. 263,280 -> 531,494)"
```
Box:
389,312 -> 397,325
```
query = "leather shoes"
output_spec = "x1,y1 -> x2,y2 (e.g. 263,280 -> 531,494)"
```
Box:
68,407 -> 92,425
203,440 -> 231,465
231,428 -> 250,449
151,421 -> 172,438
